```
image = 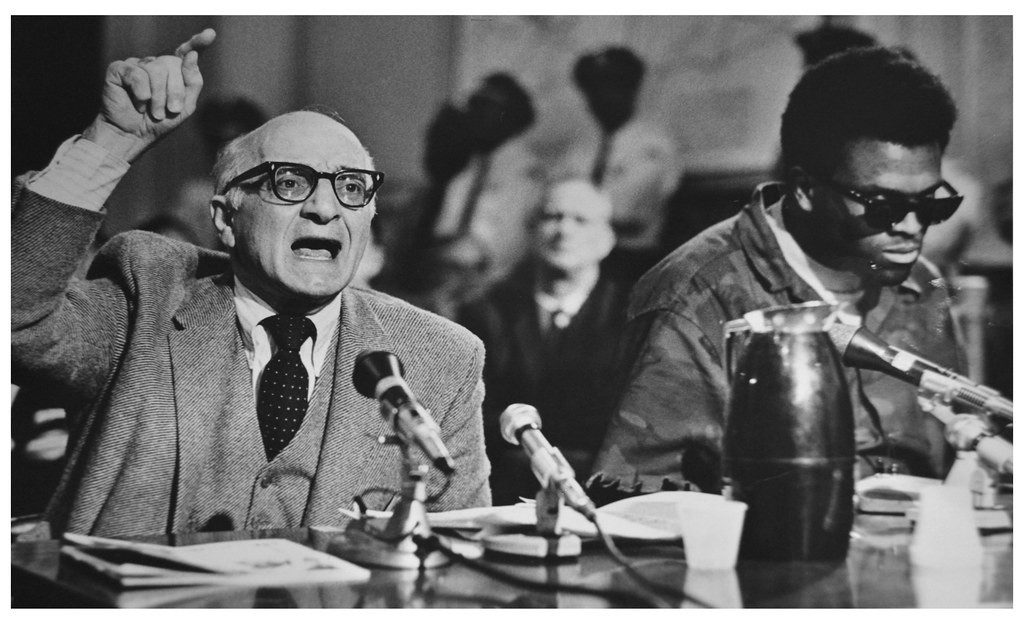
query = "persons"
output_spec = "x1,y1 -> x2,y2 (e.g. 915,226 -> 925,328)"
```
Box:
455,173 -> 645,500
584,41 -> 972,507
386,102 -> 474,317
434,70 -> 563,371
174,94 -> 273,256
559,46 -> 685,284
11,26 -> 439,541
798,29 -> 982,273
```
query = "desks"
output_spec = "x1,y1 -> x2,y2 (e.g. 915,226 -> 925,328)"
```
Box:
11,484 -> 1015,609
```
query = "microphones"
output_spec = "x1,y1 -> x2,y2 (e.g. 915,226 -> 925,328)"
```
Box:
353,346 -> 458,477
499,402 -> 599,520
829,323 -> 1014,422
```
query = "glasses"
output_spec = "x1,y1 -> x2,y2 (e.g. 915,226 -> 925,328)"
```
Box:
813,173 -> 962,228
216,160 -> 385,209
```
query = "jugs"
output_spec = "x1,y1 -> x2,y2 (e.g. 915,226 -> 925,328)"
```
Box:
715,298 -> 859,567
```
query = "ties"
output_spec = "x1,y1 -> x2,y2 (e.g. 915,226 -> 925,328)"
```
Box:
459,158 -> 490,233
255,315 -> 315,464
541,311 -> 564,356
593,134 -> 611,188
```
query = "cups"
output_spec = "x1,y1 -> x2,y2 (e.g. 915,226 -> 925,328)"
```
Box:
680,501 -> 749,569
